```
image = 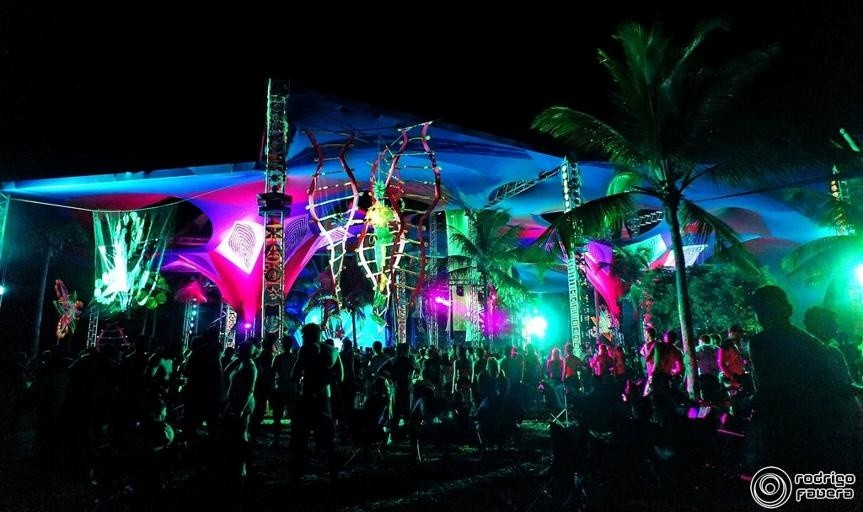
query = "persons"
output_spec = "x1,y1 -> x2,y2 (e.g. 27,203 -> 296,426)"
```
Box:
0,283 -> 863,512
